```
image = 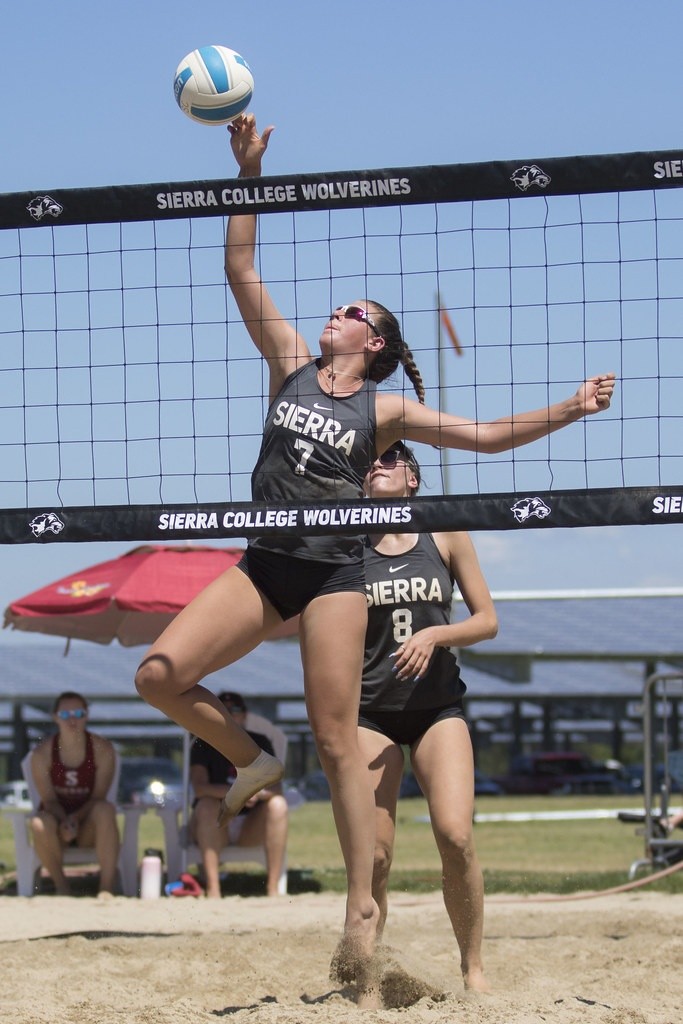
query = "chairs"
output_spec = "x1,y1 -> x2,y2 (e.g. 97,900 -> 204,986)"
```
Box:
159,714 -> 287,897
14,736 -> 140,897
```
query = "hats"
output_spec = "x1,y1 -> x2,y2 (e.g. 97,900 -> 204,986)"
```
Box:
219,692 -> 244,705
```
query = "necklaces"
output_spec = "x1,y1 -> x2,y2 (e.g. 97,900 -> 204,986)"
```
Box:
316,370 -> 362,397
321,360 -> 362,382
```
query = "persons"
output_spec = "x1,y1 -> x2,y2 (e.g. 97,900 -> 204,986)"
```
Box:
189,689 -> 288,899
354,435 -> 499,1010
136,109 -> 623,971
28,691 -> 123,902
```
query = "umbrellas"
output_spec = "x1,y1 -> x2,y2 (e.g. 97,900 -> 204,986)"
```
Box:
5,543 -> 250,827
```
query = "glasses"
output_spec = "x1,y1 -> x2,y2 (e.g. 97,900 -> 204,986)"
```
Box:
378,448 -> 408,465
225,705 -> 244,712
57,708 -> 86,719
336,305 -> 380,337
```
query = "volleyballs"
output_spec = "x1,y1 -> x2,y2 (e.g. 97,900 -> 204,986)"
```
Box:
175,46 -> 252,124
